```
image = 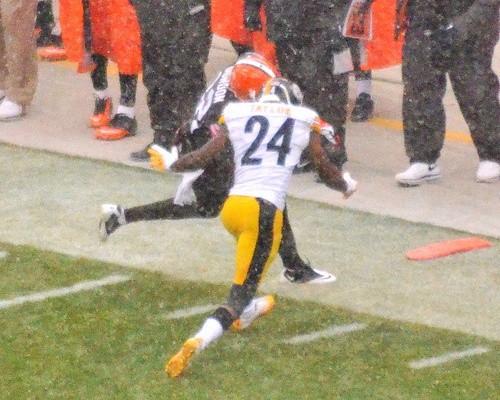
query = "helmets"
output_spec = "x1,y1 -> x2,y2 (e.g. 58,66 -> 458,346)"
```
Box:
258,78 -> 303,107
229,52 -> 282,102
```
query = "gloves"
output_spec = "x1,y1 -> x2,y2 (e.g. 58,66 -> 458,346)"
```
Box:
342,171 -> 357,194
148,143 -> 181,171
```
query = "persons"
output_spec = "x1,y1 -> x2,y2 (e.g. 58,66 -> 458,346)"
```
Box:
98,53 -> 336,284
147,79 -> 359,377
1,0 -> 500,198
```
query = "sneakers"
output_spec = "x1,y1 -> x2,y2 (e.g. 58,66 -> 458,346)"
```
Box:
130,142 -> 155,161
0,90 -> 5,100
476,161 -> 500,182
232,295 -> 275,330
89,94 -> 113,128
350,93 -> 374,122
36,34 -> 68,62
293,127 -> 347,174
0,100 -> 25,120
165,338 -> 202,379
94,113 -> 136,141
280,265 -> 336,284
33,0 -> 54,40
99,204 -> 126,242
395,162 -> 440,186
